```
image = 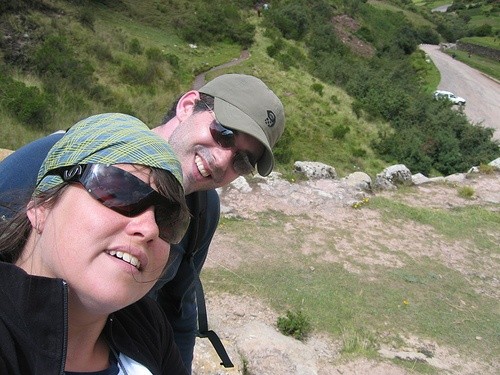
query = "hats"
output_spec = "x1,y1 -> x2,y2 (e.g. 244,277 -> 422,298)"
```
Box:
199,75 -> 285,176
36,113 -> 185,198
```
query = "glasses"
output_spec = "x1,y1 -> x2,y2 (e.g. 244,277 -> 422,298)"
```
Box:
37,164 -> 190,245
201,99 -> 255,177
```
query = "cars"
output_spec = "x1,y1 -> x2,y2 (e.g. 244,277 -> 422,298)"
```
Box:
433,91 -> 467,106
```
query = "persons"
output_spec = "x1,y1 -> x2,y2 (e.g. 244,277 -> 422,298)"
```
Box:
0,73 -> 287,375
0,111 -> 192,375
